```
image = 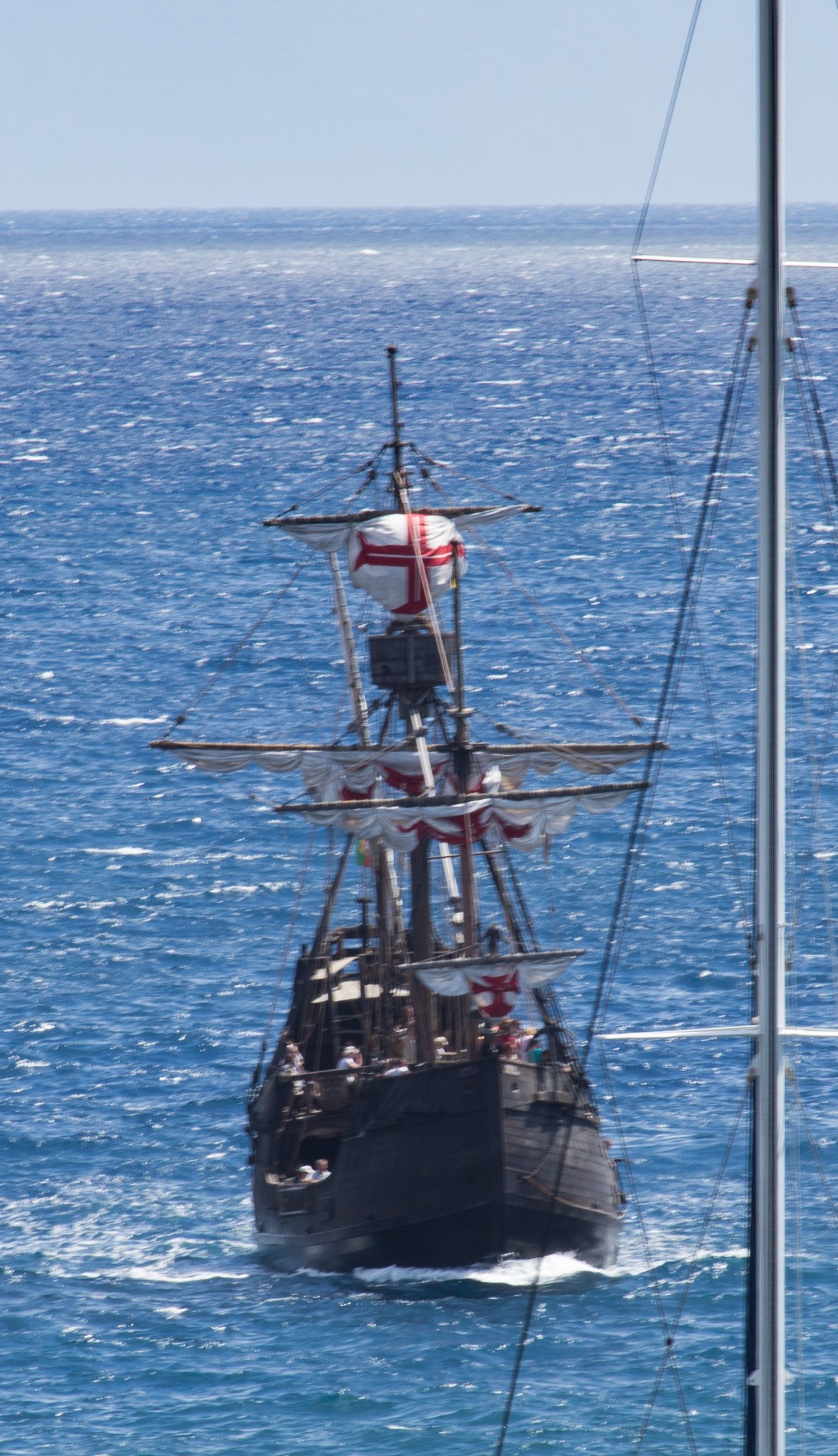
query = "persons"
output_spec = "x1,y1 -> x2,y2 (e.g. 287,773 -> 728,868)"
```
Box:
283,1164 -> 319,1186
274,1007 -> 558,1112
306,1158 -> 333,1183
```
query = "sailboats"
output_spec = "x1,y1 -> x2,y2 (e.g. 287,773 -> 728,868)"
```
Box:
148,346 -> 671,1262
